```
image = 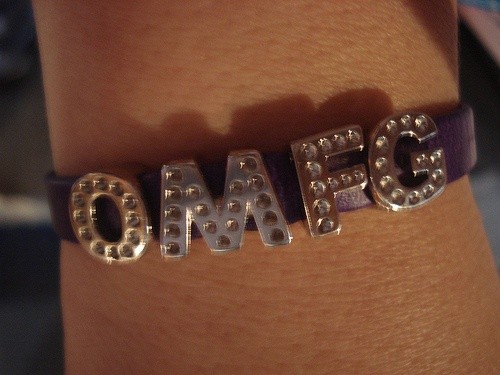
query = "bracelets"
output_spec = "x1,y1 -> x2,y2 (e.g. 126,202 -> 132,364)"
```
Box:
44,103 -> 480,266
